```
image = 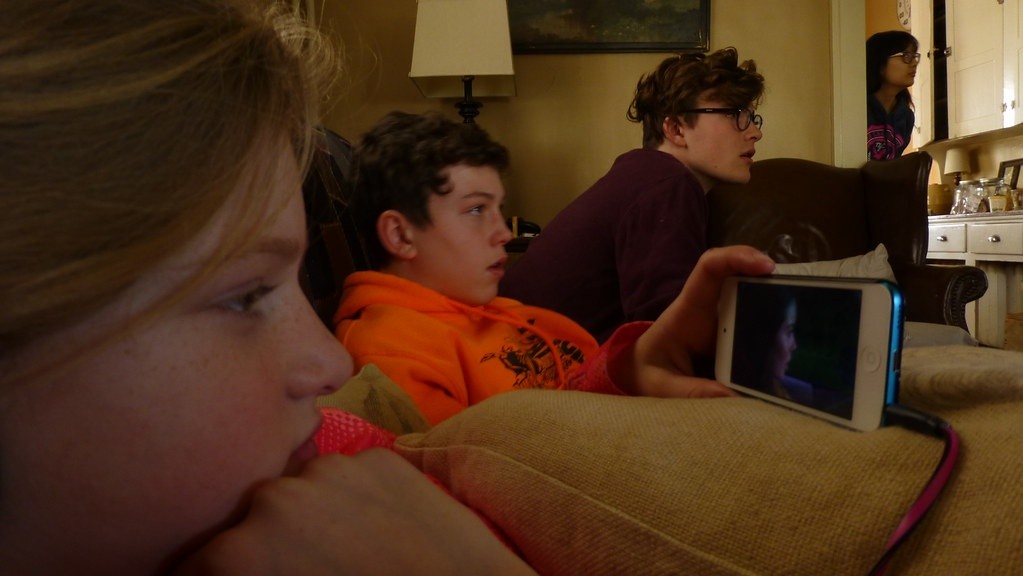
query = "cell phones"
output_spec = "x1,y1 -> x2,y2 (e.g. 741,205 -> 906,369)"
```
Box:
713,273 -> 905,431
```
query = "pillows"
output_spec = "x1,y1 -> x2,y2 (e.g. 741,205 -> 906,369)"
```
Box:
391,347 -> 1023,576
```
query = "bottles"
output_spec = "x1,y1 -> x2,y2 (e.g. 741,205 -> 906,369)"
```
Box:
953,179 -> 985,215
928,183 -> 953,216
976,177 -> 1006,202
988,196 -> 1006,213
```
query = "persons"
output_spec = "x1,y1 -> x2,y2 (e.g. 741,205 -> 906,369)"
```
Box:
498,45 -> 763,349
1,1 -> 776,575
867,30 -> 919,163
733,292 -> 799,406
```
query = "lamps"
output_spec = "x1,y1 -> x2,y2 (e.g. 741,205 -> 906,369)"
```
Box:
943,148 -> 971,186
406,0 -> 516,123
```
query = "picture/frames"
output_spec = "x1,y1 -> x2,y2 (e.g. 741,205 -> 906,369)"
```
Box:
507,0 -> 711,53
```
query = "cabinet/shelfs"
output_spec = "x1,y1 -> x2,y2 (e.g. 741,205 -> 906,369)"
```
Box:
909,0 -> 1023,150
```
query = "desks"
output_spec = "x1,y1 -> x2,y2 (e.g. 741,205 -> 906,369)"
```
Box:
926,211 -> 1023,345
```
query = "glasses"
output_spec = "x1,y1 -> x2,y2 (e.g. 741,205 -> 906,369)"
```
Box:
889,50 -> 921,65
672,102 -> 763,132
